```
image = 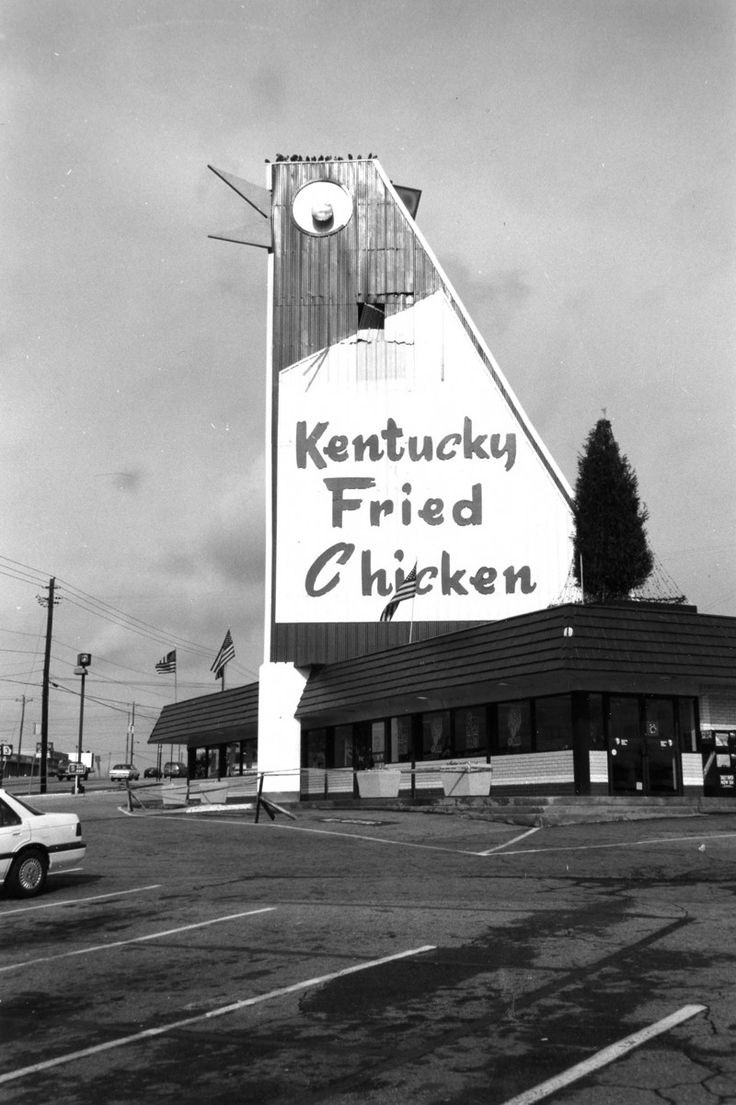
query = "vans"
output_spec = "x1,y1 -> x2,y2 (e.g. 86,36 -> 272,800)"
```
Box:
164,762 -> 186,778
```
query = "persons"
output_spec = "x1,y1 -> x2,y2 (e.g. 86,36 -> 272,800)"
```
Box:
195,753 -> 206,779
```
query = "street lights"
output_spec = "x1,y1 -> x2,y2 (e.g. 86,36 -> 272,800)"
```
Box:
71,653 -> 92,794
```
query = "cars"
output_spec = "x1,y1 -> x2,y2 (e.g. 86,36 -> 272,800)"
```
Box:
0,789 -> 87,898
109,764 -> 140,782
144,767 -> 163,778
57,760 -> 88,781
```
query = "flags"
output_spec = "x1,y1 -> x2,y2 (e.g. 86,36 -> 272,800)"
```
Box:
210,630 -> 235,680
155,650 -> 176,675
